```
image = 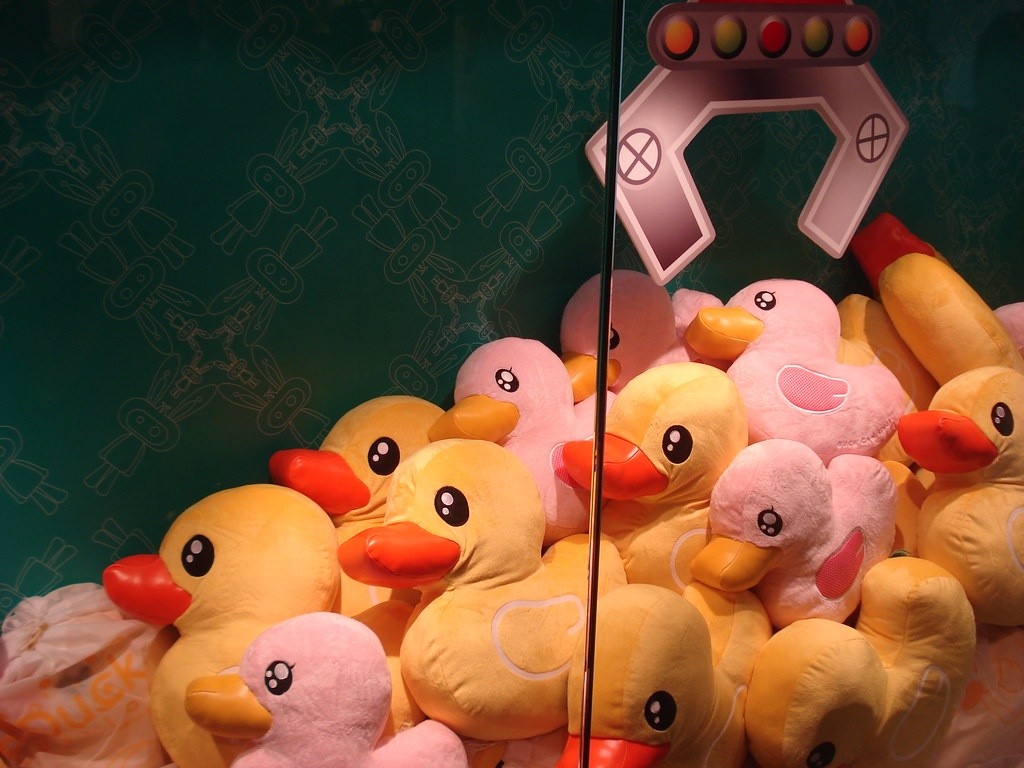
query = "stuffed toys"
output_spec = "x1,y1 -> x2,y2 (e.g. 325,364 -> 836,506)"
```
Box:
102,212 -> 1024,768
